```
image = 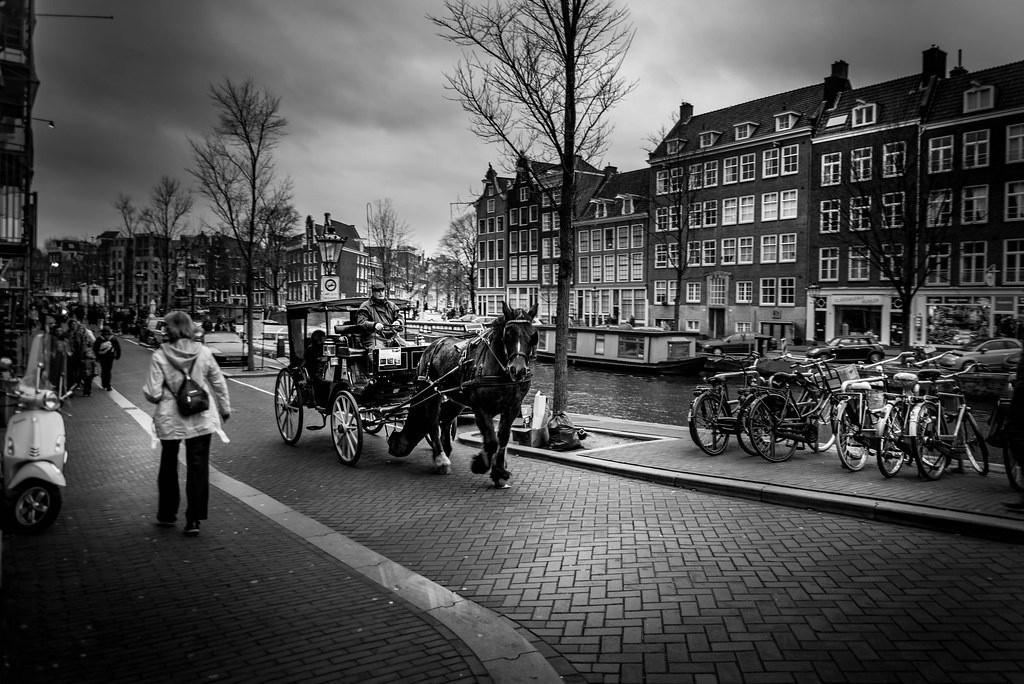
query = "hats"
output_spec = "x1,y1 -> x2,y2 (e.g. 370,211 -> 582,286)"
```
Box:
372,280 -> 385,290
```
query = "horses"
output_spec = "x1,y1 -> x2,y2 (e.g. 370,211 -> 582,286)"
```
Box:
410,300 -> 539,488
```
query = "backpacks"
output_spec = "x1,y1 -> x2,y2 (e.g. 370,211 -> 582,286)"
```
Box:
162,354 -> 209,416
99,334 -> 116,358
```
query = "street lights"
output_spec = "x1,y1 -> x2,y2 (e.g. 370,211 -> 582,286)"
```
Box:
185,255 -> 202,322
316,222 -> 348,333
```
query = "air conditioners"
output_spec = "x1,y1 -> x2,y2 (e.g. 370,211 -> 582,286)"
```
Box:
770,308 -> 781,319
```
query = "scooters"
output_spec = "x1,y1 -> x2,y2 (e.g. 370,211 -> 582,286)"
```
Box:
0,356 -> 78,537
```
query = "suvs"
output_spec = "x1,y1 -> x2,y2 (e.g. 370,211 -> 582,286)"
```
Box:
804,335 -> 885,364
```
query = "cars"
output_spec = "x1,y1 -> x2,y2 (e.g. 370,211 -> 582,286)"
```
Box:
939,336 -> 1024,374
704,331 -> 777,356
443,313 -> 547,327
141,316 -> 249,367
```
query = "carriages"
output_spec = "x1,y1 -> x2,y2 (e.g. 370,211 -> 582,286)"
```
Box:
273,296 -> 538,489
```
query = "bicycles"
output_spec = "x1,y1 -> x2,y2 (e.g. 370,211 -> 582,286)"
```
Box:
686,351 -> 990,482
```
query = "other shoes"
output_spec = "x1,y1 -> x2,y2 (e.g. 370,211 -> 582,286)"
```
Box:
157,511 -> 177,523
103,383 -> 113,391
84,393 -> 92,397
184,518 -> 199,534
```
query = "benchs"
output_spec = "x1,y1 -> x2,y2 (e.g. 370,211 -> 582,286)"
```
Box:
334,325 -> 421,358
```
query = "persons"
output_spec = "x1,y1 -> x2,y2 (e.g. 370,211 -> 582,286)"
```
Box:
811,336 -> 818,346
424,303 -> 428,310
201,315 -> 235,332
143,310 -> 231,532
629,315 -> 635,327
358,283 -> 405,359
447,305 -> 464,319
605,314 -> 617,325
27,297 -> 136,334
306,330 -> 326,374
50,318 -> 121,396
